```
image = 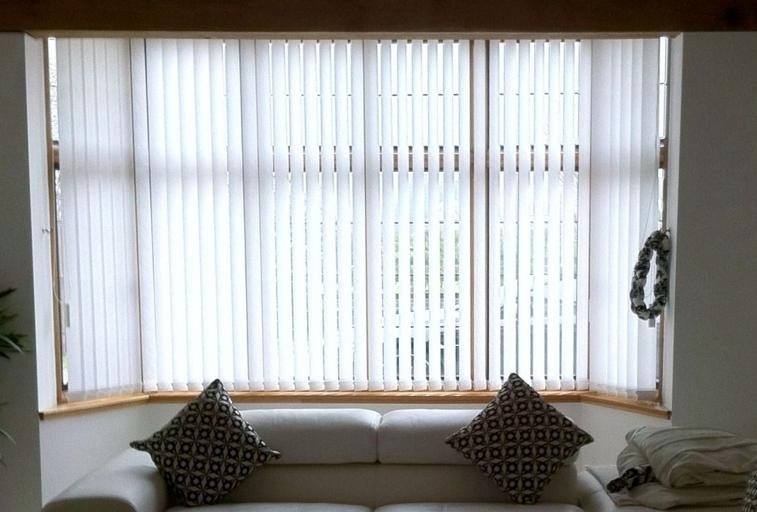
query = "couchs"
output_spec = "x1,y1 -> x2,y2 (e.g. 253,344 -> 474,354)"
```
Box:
40,409 -> 617,512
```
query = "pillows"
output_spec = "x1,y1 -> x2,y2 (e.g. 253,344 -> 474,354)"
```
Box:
128,378 -> 282,508
443,373 -> 594,505
